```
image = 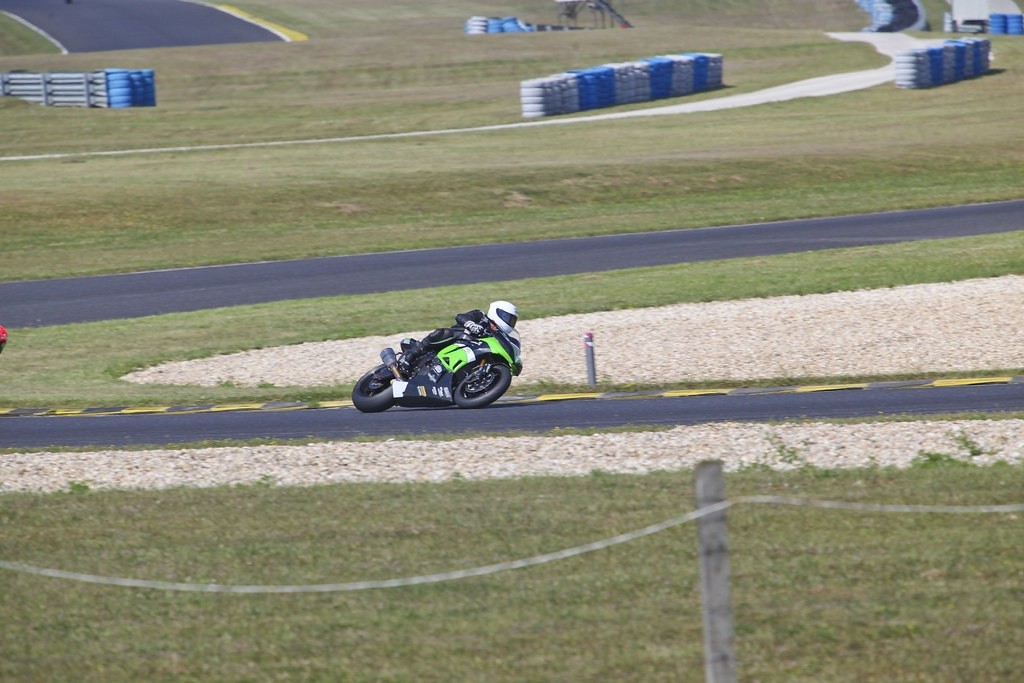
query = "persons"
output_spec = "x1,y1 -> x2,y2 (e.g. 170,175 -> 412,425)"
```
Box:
405,296 -> 524,376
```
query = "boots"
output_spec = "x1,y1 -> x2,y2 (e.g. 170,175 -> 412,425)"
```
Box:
399,341 -> 426,379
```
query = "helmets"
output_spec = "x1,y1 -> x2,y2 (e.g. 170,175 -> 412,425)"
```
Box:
486,300 -> 518,335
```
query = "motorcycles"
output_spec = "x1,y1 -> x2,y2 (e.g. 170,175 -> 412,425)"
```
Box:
351,319 -> 521,412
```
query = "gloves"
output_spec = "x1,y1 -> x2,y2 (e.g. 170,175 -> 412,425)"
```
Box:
463,320 -> 484,334
